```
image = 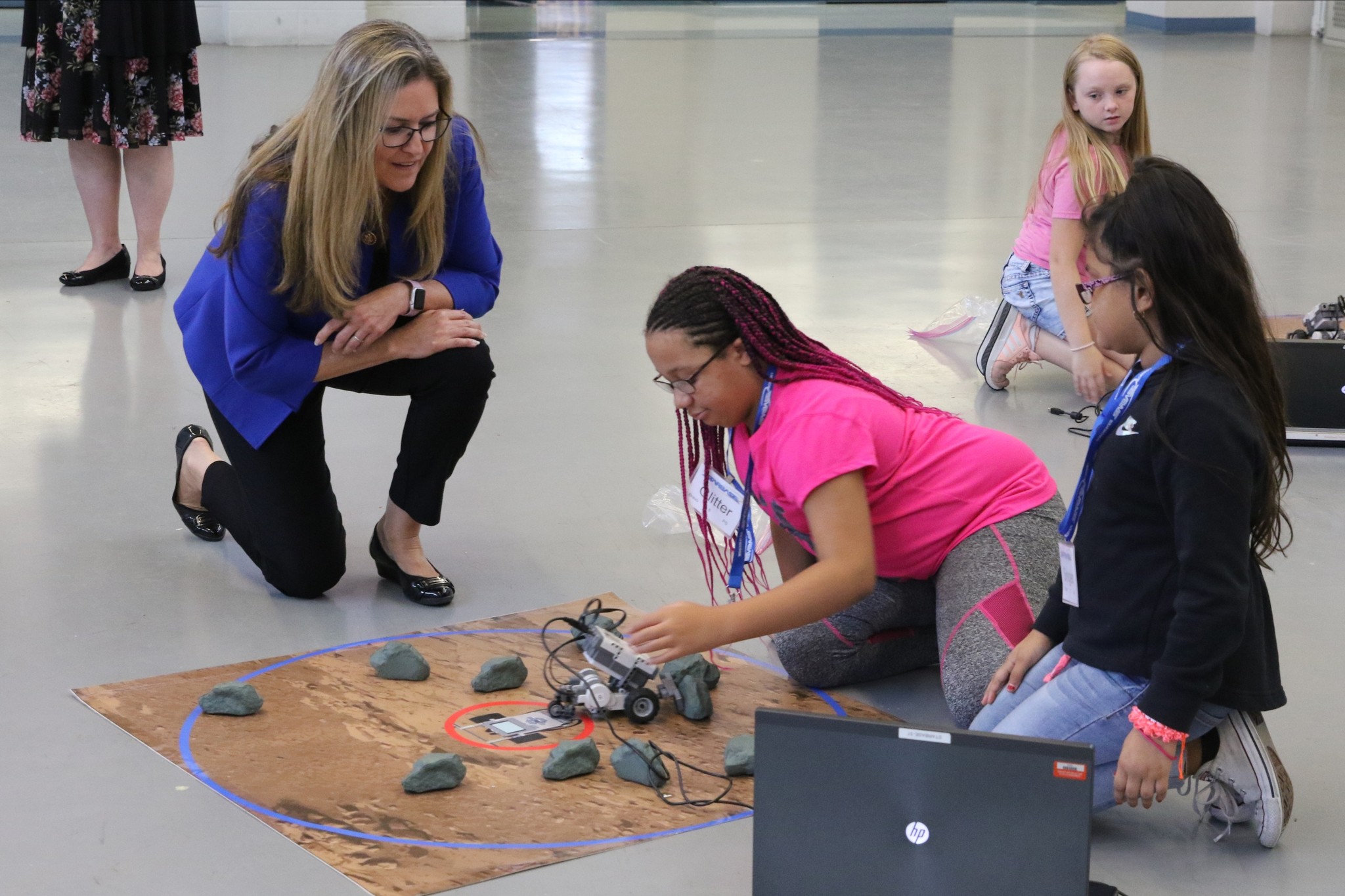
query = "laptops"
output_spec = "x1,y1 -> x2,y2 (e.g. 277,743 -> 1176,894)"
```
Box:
751,707 -> 1117,896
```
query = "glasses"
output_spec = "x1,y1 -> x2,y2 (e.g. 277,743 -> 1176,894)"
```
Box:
652,338 -> 737,398
379,107 -> 453,148
1074,270 -> 1131,305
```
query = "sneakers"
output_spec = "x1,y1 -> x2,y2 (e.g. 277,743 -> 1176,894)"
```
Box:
982,302 -> 1043,391
976,298 -> 1007,377
1194,775 -> 1259,825
1193,707 -> 1294,850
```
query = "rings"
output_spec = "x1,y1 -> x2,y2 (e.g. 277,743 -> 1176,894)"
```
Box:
353,335 -> 365,343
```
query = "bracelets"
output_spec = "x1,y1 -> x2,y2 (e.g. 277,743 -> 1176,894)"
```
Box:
1137,729 -> 1177,761
1129,706 -> 1189,780
1070,341 -> 1096,351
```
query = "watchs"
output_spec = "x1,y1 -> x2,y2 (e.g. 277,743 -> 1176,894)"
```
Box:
398,279 -> 426,317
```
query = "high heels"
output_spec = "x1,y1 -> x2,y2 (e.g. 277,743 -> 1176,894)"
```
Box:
173,424 -> 226,542
59,243 -> 131,286
130,252 -> 166,291
369,521 -> 456,607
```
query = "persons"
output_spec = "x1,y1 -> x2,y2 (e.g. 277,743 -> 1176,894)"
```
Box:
974,33 -> 1150,409
170,19 -> 502,608
968,155 -> 1296,848
19,0 -> 204,292
621,264 -> 1066,730
